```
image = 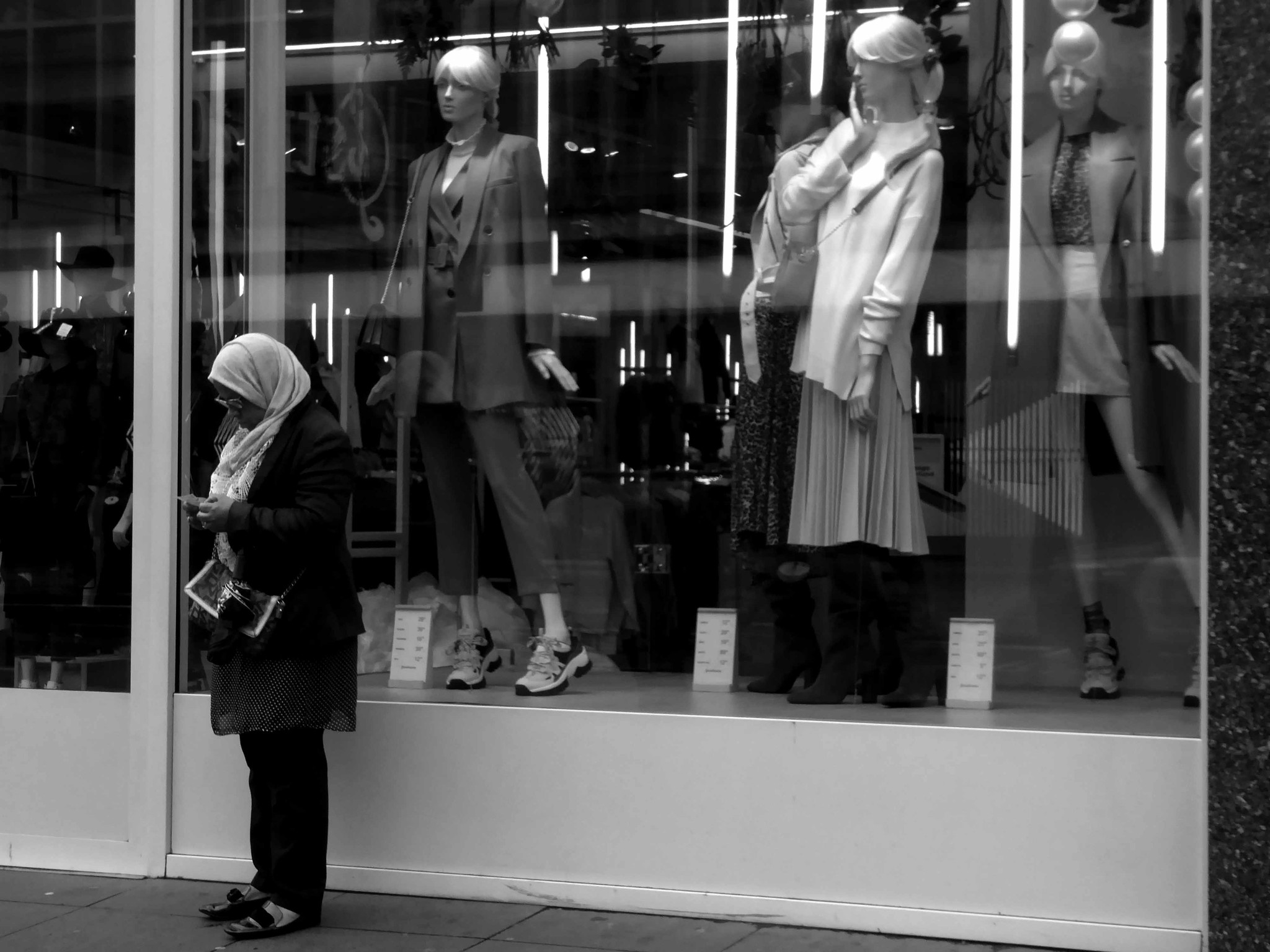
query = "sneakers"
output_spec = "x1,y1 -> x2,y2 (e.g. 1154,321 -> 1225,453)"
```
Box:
446,627 -> 503,691
514,625 -> 594,696
1080,632 -> 1125,699
1183,655 -> 1200,707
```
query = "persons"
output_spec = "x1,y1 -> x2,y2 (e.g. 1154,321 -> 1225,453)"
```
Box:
182,336 -> 364,939
367,42 -> 592,696
730,15 -> 943,703
0,246 -> 132,692
1006,20 -> 1205,708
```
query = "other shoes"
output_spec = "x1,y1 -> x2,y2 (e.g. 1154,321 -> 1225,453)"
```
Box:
223,900 -> 322,940
199,885 -> 273,921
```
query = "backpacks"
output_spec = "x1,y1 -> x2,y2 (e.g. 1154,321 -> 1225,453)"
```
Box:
616,375 -> 682,468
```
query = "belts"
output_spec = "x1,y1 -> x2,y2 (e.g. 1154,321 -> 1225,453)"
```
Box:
427,243 -> 455,269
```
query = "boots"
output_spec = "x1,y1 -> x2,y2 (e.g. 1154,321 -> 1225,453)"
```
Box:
747,564 -> 823,693
788,546 -> 878,706
873,553 -> 947,707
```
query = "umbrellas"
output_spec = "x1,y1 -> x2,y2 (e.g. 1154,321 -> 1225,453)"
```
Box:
208,578 -> 256,664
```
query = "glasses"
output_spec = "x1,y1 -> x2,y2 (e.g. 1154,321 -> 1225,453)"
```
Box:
214,393 -> 247,411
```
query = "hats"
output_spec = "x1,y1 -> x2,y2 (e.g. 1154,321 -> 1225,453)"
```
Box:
56,245 -> 127,292
18,307 -> 95,359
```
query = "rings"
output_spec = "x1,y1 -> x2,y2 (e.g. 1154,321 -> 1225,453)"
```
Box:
202,521 -> 206,528
187,516 -> 190,524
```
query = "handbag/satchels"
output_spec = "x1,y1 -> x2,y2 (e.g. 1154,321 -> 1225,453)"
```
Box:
357,303 -> 401,357
183,558 -> 286,655
